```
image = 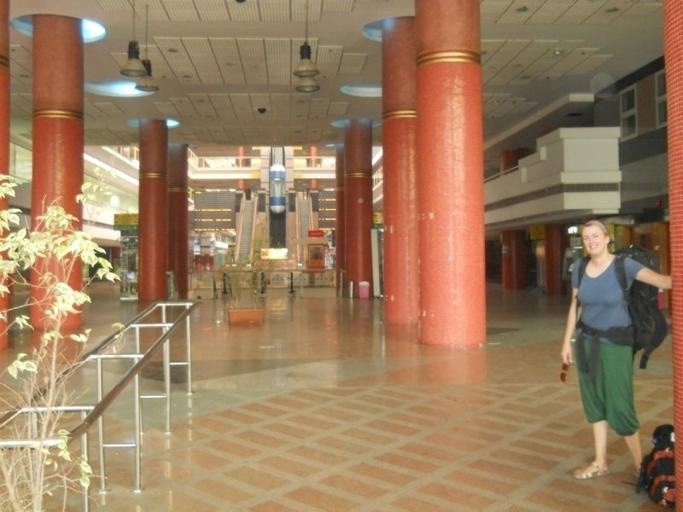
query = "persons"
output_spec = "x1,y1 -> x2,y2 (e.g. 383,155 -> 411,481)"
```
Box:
560,218 -> 672,479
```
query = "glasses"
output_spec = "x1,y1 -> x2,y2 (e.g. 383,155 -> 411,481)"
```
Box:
558,360 -> 588,387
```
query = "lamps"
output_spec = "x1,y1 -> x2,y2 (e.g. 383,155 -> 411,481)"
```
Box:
294,76 -> 320,93
135,3 -> 160,92
294,0 -> 320,77
119,0 -> 147,78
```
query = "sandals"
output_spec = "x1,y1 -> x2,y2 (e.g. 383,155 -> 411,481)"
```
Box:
572,461 -> 609,480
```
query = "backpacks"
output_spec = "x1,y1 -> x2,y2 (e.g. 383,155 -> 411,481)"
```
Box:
574,245 -> 666,349
635,421 -> 677,507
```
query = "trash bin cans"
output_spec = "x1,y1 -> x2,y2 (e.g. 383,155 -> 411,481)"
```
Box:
359,281 -> 369,299
